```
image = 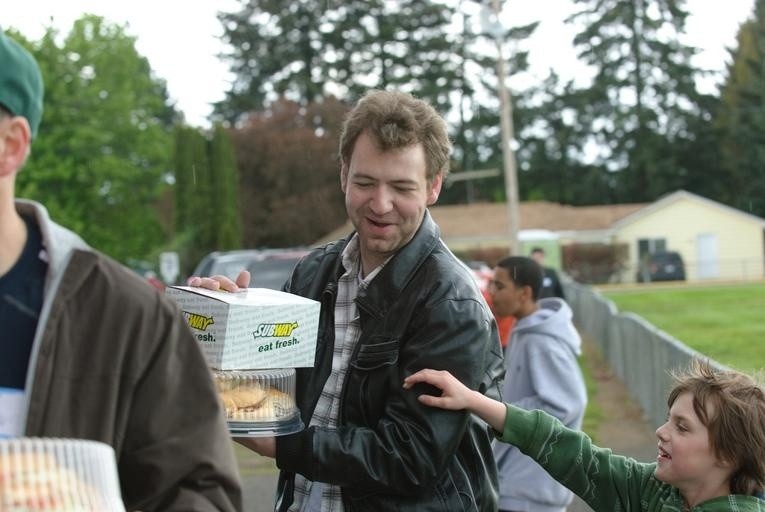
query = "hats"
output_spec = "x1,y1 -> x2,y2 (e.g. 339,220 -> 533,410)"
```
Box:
0,34 -> 46,138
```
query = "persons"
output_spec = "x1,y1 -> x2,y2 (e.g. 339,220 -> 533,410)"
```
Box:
471,257 -> 586,512
474,271 -> 513,354
0,30 -> 245,512
530,249 -> 566,301
404,358 -> 765,512
187,88 -> 506,512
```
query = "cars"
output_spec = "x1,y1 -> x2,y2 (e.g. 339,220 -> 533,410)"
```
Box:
190,247 -> 324,287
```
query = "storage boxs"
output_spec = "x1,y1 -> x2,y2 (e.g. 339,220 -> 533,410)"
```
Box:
165,286 -> 322,372
